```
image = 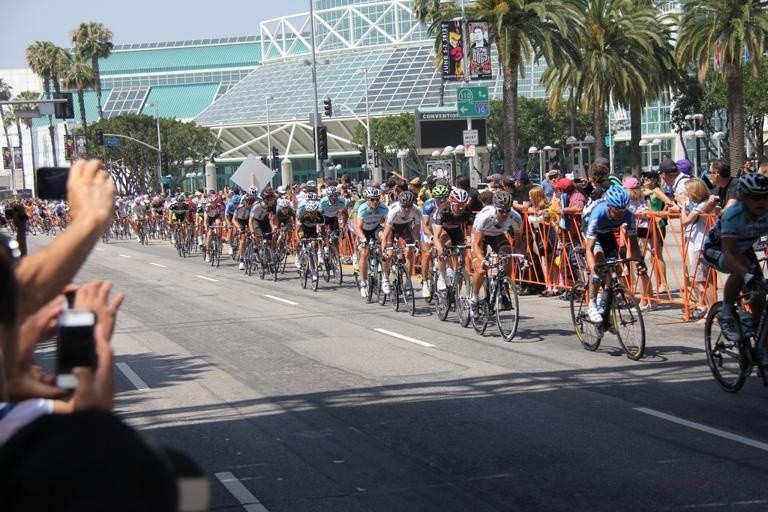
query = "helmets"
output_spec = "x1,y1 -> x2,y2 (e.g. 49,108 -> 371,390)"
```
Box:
261,189 -> 276,200
304,201 -> 320,212
326,185 -> 341,196
306,180 -> 317,187
492,191 -> 512,210
248,185 -> 259,195
449,189 -> 469,205
208,194 -> 217,202
737,173 -> 768,199
201,198 -> 209,203
398,191 -> 416,205
117,198 -> 124,203
304,192 -> 316,200
232,195 -> 241,204
606,185 -> 630,208
364,187 -> 381,198
175,195 -> 185,203
432,185 -> 449,198
135,199 -> 141,204
279,199 -> 290,207
245,196 -> 255,204
195,191 -> 202,196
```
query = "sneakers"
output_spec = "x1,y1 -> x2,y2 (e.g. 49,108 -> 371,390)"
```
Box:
381,278 -> 392,296
405,282 -> 413,296
323,247 -> 331,260
228,247 -> 233,255
351,255 -> 359,271
205,253 -> 212,263
446,266 -> 454,278
690,307 -> 706,319
588,305 -> 603,323
560,292 -> 567,300
499,293 -> 514,311
422,280 -> 431,298
553,288 -> 560,295
627,302 -> 649,312
317,251 -> 324,264
197,237 -> 203,246
649,302 -> 658,311
716,312 -> 741,341
469,299 -> 480,319
436,274 -> 446,291
542,289 -> 553,296
359,282 -> 370,298
239,261 -> 245,270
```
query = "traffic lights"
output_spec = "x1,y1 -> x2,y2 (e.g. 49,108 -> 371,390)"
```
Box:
94,128 -> 106,148
312,126 -> 327,161
324,98 -> 332,119
261,156 -> 268,172
271,144 -> 281,172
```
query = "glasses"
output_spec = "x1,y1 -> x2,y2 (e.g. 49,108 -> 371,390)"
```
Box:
401,204 -> 413,208
750,195 -> 767,200
370,198 -> 380,201
497,209 -> 511,213
549,176 -> 558,180
707,169 -> 720,174
643,175 -> 652,179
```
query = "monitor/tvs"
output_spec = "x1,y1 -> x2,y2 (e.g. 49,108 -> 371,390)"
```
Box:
415,107 -> 487,155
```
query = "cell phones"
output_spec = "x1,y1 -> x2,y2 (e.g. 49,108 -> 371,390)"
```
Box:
57,312 -> 96,388
38,167 -> 68,202
574,179 -> 581,184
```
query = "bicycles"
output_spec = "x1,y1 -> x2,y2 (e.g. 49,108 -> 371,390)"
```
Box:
568,256 -> 647,363
295,238 -> 319,291
351,242 -> 390,311
388,242 -> 422,316
702,256 -> 767,394
320,234 -> 344,287
433,244 -> 471,329
0,208 -> 290,282
472,252 -> 530,341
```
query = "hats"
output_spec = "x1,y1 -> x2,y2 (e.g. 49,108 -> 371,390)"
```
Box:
623,177 -> 640,188
510,169 -> 527,181
641,171 -> 659,178
410,177 -> 421,185
656,160 -> 678,174
549,169 -> 561,177
487,173 -> 502,181
427,175 -> 438,184
277,186 -> 287,193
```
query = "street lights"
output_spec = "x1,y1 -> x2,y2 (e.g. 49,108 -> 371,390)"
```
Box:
710,132 -> 727,163
104,102 -> 164,193
639,136 -> 662,172
526,146 -> 554,183
684,112 -> 705,179
431,144 -> 467,186
327,163 -> 342,184
564,135 -> 594,181
360,163 -> 370,187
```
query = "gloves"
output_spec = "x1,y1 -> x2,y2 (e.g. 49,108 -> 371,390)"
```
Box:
482,261 -> 491,271
591,273 -> 601,285
743,273 -> 759,286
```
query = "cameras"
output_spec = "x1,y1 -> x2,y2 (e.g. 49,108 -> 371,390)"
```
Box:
5,207 -> 27,219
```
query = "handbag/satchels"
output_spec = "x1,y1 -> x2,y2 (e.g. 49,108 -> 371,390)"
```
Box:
636,213 -> 651,238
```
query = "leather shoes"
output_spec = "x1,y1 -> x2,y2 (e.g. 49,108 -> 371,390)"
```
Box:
519,288 -> 538,295
658,283 -> 668,294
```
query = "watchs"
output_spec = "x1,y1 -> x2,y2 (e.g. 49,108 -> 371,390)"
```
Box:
430,236 -> 434,240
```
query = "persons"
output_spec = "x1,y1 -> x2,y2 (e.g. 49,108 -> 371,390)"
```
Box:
757,155 -> 768,177
294,200 -> 325,269
547,169 -> 563,188
375,189 -> 426,300
316,186 -> 349,260
656,159 -> 694,198
622,177 -> 658,312
702,173 -> 768,341
0,184 -> 201,252
736,158 -> 754,179
638,170 -> 675,292
586,163 -> 615,206
407,177 -> 432,208
224,194 -> 241,255
1,156 -> 119,327
231,194 -> 256,270
528,187 -> 558,296
378,191 -> 422,294
673,179 -> 720,319
470,25 -> 490,48
663,153 -> 670,161
203,193 -> 228,262
347,187 -> 369,270
585,185 -> 648,322
708,158 -> 742,214
0,253 -> 125,446
700,160 -> 714,179
210,170 -> 408,202
248,188 -> 278,263
275,199 -> 297,256
595,157 -> 623,185
469,190 -> 532,307
355,187 -> 394,298
541,173 -> 554,201
556,179 -> 586,218
419,184 -> 458,298
511,166 -> 538,297
430,189 -> 475,299
